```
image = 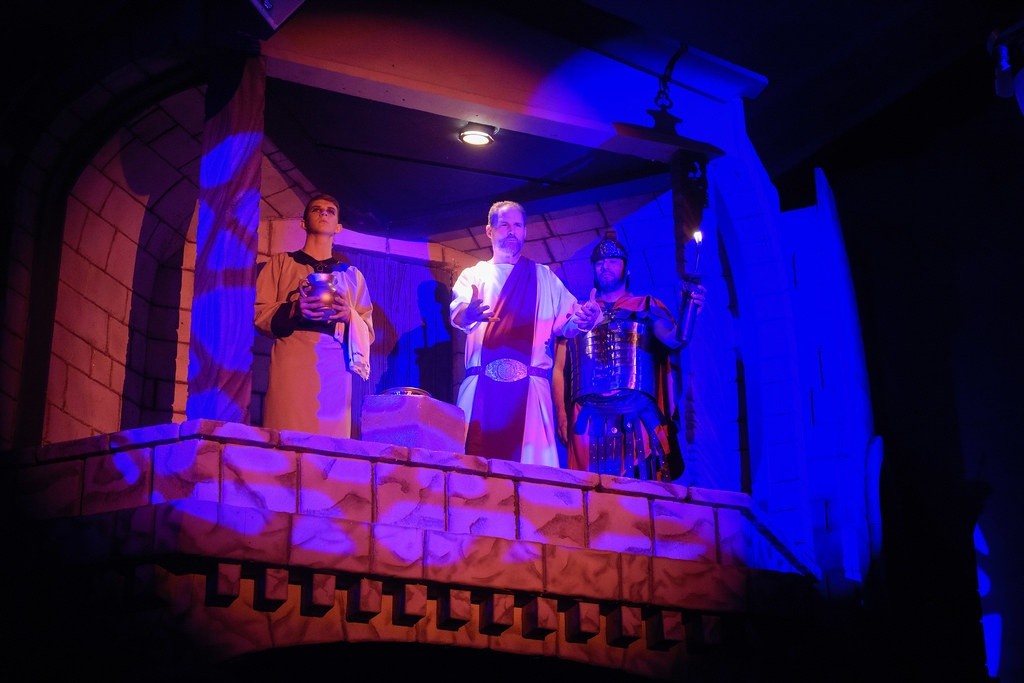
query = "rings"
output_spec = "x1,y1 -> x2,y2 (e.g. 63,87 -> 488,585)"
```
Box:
584,315 -> 589,321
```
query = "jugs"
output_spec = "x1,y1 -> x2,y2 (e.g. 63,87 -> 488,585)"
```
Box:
297,272 -> 343,321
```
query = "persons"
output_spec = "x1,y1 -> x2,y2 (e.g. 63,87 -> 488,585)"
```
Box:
549,233 -> 707,483
449,201 -> 604,465
250,192 -> 375,442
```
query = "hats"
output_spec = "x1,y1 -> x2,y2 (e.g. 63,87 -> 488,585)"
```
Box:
591,229 -> 627,262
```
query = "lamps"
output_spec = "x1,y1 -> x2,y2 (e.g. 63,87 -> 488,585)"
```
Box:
458,122 -> 500,146
